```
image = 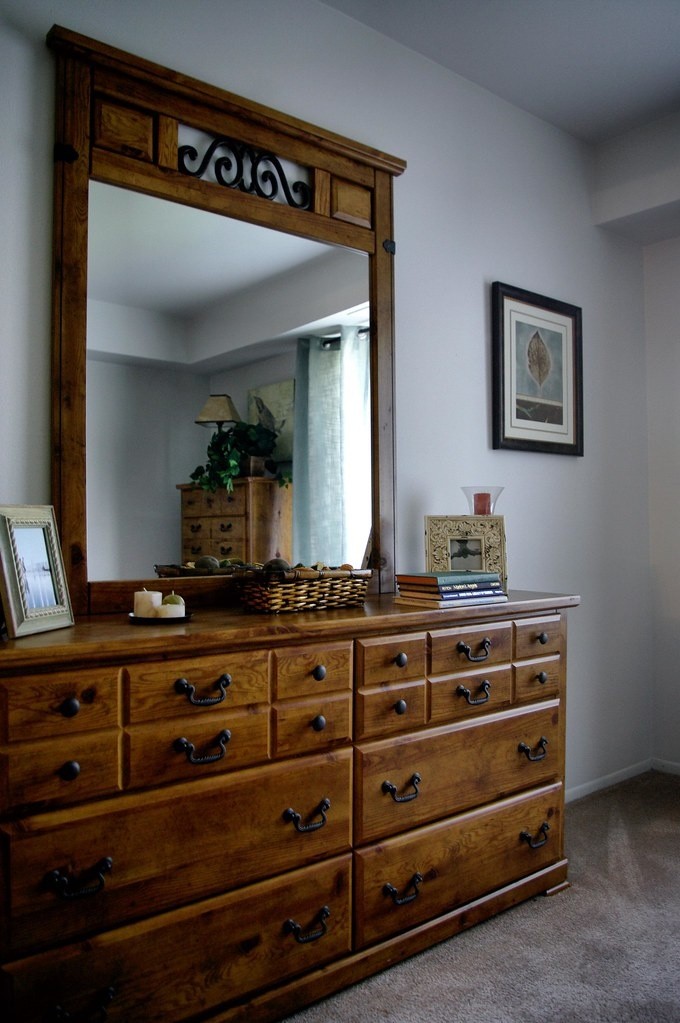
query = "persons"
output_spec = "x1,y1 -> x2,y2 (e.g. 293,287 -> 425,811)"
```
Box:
19,558 -> 30,595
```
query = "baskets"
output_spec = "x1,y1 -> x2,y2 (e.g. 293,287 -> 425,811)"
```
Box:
232,569 -> 372,614
154,565 -> 242,577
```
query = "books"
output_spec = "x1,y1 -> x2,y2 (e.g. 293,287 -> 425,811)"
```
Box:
392,572 -> 508,609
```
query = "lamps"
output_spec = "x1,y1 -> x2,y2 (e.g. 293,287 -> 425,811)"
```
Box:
195,393 -> 240,435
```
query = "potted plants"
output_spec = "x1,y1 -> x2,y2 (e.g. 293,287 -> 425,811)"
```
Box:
189,421 -> 292,497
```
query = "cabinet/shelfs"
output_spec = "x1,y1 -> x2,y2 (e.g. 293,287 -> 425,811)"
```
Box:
175,476 -> 293,565
1,590 -> 580,1023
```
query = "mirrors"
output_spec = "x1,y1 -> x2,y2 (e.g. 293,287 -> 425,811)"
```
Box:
44,22 -> 408,612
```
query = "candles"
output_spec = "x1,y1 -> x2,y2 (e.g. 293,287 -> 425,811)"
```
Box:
134,590 -> 163,617
159,605 -> 185,617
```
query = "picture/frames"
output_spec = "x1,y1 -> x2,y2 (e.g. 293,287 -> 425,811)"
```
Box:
423,513 -> 509,594
490,281 -> 585,457
0,503 -> 76,637
